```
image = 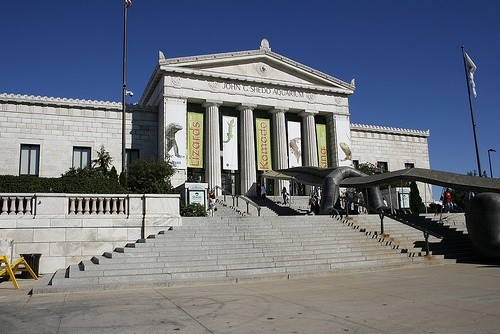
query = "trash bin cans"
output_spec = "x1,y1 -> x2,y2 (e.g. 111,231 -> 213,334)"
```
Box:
19,254 -> 42,279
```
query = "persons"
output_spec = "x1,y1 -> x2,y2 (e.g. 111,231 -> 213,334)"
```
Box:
280,187 -> 290,205
208,190 -> 216,211
443,189 -> 453,213
309,194 -> 320,213
341,188 -> 389,214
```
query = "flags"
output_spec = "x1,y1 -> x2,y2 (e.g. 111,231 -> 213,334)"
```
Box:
462,50 -> 478,98
125,0 -> 132,8
